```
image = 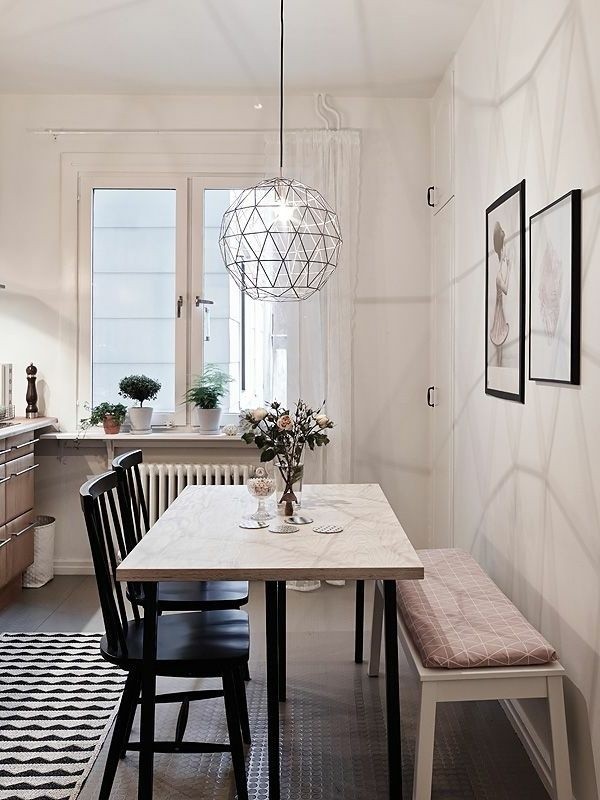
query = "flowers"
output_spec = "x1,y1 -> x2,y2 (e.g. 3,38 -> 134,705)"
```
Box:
242,396 -> 336,503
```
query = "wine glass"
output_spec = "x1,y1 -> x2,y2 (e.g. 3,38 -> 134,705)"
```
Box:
246,460 -> 277,521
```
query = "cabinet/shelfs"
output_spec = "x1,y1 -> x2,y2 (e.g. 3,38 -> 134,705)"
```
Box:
0,431 -> 40,614
425,69 -> 455,549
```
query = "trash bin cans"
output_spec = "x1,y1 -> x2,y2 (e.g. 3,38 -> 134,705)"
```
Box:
22,515 -> 56,588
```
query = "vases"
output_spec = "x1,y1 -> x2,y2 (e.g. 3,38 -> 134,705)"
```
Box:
273,442 -> 305,514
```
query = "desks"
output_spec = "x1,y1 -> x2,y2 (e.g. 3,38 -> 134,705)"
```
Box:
116,483 -> 425,800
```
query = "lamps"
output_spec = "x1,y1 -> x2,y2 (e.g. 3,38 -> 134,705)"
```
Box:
219,0 -> 343,303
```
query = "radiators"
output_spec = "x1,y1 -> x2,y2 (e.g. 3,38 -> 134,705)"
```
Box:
125,464 -> 257,552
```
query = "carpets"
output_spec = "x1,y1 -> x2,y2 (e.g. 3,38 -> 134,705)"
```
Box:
0,632 -> 129,800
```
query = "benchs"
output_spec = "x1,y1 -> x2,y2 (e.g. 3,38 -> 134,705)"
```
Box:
367,550 -> 575,800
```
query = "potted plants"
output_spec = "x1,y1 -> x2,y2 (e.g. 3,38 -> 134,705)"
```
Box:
118,374 -> 162,430
71,402 -> 129,450
179,362 -> 235,432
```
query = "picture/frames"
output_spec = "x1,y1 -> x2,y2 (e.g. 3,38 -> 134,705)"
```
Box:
529,188 -> 582,384
484,179 -> 526,404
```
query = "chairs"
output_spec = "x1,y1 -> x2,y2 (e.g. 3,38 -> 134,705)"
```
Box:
79,449 -> 252,800
